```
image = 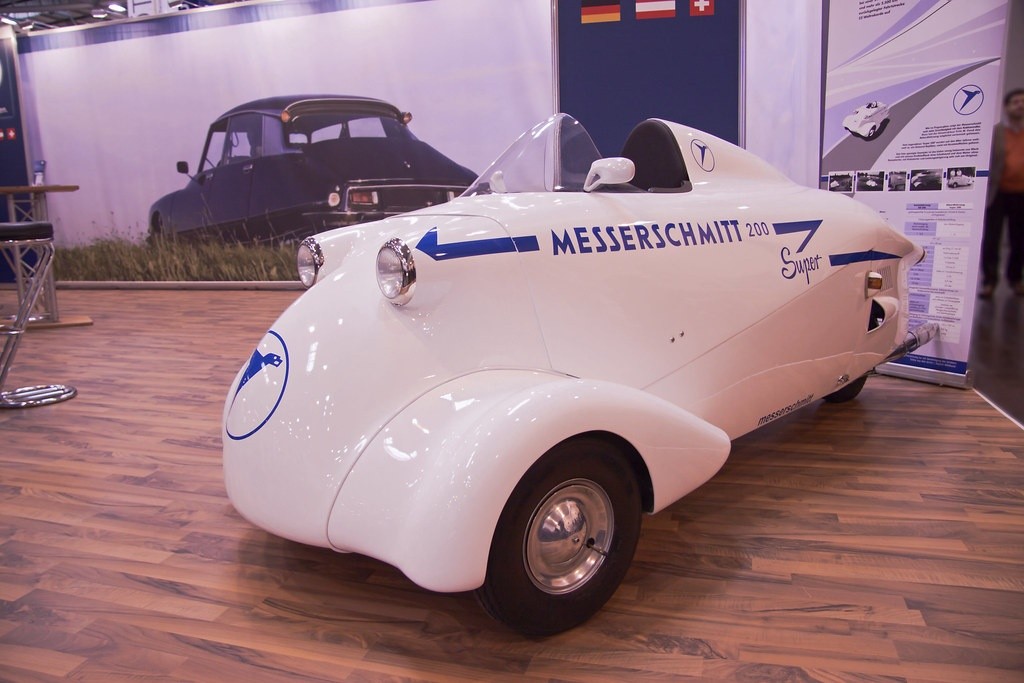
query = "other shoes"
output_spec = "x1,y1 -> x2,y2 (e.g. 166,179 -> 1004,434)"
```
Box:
979,287 -> 995,300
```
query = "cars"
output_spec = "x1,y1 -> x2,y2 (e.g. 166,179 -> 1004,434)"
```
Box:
842,100 -> 889,140
223,113 -> 940,640
146,94 -> 493,247
948,174 -> 973,188
911,170 -> 941,191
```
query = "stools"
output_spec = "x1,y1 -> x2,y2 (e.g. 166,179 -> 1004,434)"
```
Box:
0,221 -> 78,408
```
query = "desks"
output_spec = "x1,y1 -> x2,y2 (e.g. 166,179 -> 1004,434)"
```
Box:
0,186 -> 93,329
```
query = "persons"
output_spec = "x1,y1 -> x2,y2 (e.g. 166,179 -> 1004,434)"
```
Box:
950,168 -> 962,177
978,89 -> 1024,299
